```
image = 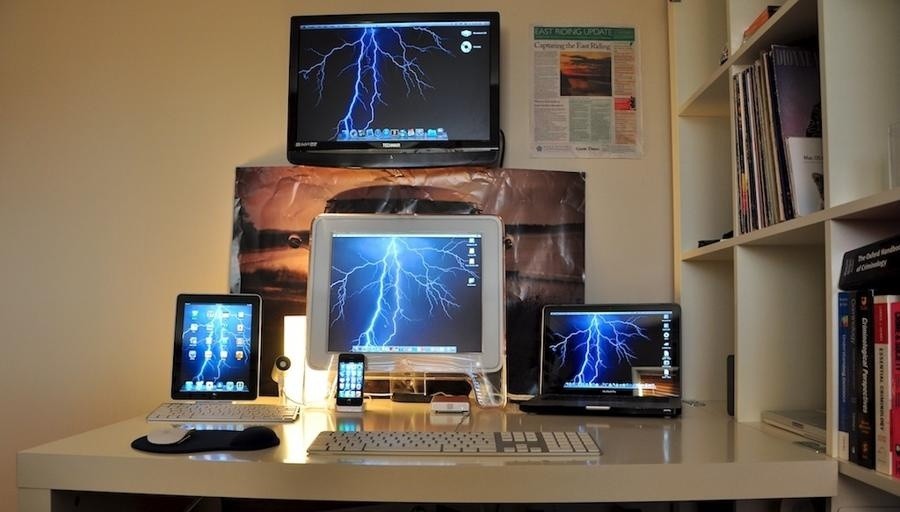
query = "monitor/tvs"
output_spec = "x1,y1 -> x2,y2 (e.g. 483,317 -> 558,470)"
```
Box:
287,11 -> 501,167
306,212 -> 507,408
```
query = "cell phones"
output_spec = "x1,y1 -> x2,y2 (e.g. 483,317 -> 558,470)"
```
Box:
335,353 -> 366,407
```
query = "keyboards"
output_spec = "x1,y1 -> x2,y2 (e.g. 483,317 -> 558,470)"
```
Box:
306,431 -> 602,458
144,403 -> 301,422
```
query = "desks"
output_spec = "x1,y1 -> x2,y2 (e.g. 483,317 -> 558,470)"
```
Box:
14,405 -> 841,512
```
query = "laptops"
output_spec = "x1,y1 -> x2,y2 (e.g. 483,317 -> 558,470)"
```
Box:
518,302 -> 683,419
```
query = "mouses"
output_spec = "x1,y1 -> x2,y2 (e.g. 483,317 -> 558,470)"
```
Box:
146,426 -> 197,447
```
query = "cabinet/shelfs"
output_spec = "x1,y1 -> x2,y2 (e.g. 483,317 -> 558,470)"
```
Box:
665,2 -> 900,510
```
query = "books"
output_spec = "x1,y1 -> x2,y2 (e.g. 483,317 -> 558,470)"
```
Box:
763,410 -> 826,446
733,5 -> 824,234
838,235 -> 900,479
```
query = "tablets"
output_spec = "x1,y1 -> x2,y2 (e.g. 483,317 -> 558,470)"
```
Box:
171,292 -> 263,402
